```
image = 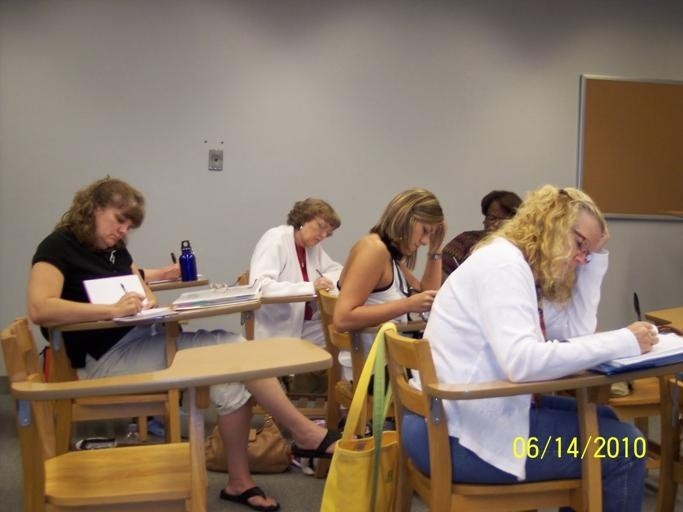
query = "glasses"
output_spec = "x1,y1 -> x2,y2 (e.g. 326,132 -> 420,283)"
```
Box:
575,228 -> 592,261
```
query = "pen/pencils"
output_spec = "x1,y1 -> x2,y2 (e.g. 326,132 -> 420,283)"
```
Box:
171,252 -> 176,263
633,292 -> 641,321
121,284 -> 142,314
316,269 -> 323,277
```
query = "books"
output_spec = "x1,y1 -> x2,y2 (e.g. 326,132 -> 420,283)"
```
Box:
82,274 -> 178,322
604,329 -> 683,368
173,285 -> 259,310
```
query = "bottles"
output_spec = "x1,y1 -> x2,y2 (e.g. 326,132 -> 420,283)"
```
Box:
177,239 -> 197,283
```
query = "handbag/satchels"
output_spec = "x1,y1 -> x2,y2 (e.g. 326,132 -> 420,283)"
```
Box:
322,431 -> 413,512
205,412 -> 293,474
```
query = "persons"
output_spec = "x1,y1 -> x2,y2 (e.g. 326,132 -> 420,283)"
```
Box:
400,185 -> 659,511
250,198 -> 344,350
27,180 -> 363,511
139,262 -> 181,284
333,188 -> 443,394
442,191 -> 524,281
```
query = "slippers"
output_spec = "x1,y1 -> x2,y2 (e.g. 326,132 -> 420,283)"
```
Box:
292,428 -> 363,459
220,483 -> 279,510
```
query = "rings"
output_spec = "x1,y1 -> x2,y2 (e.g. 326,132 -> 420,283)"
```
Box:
326,288 -> 330,291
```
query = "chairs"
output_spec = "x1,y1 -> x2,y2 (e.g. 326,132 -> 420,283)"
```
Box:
378,320 -> 683,510
313,288 -> 427,481
2,273 -> 336,509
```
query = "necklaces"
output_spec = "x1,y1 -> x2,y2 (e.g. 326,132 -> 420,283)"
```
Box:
297,246 -> 306,268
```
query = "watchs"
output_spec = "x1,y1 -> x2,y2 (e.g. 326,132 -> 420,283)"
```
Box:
427,253 -> 441,260
